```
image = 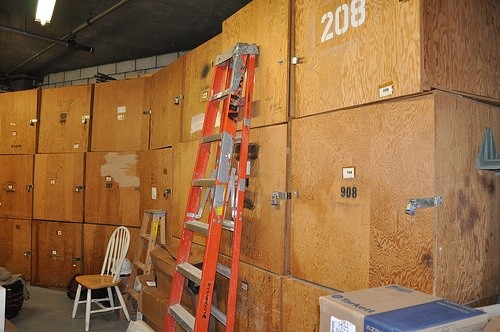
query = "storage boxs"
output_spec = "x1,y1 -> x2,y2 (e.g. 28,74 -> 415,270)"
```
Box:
319,283 -> 490,332
139,244 -> 217,332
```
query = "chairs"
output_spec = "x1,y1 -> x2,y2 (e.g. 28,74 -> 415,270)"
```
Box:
71,225 -> 131,331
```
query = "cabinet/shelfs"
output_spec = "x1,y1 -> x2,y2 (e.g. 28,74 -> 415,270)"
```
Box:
185,0 -> 500,332
0,54 -> 184,290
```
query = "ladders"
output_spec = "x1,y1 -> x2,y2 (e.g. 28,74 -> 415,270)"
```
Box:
120,210 -> 166,321
164,43 -> 259,332
